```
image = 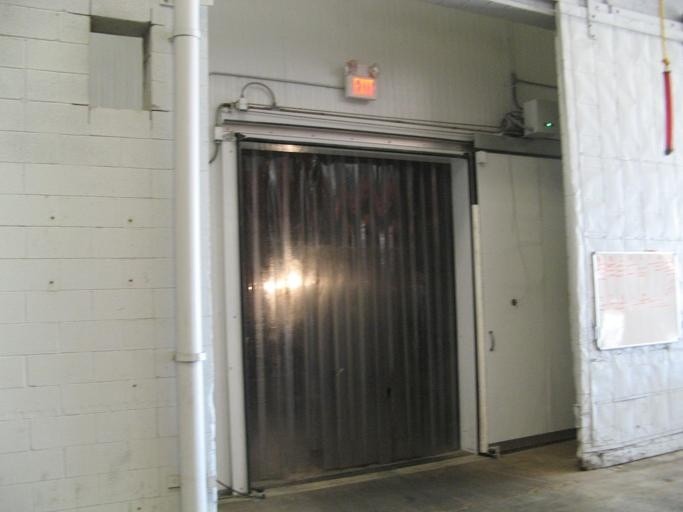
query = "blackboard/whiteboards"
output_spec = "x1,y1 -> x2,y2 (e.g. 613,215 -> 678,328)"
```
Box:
592,251 -> 682,351
